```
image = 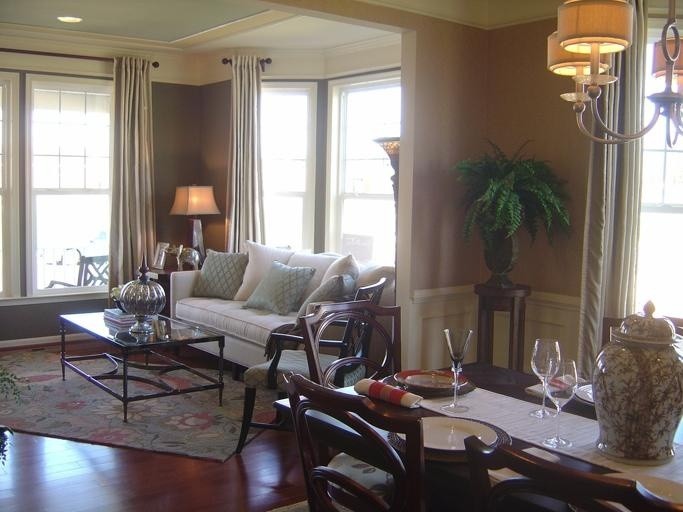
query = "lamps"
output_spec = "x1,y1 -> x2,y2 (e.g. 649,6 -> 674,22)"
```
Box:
372,137 -> 400,305
547,0 -> 683,149
168,184 -> 222,269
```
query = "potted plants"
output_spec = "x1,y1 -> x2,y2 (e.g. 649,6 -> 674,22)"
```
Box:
445,137 -> 571,290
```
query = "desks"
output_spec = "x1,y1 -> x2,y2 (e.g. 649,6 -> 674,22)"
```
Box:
277,361 -> 681,509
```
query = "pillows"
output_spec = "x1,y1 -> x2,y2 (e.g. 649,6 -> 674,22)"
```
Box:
193,238 -> 394,323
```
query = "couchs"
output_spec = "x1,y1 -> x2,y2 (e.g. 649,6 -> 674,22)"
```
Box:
170,269 -> 398,382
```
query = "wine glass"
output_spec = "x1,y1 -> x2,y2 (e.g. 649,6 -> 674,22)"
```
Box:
442,329 -> 472,414
530,338 -> 578,450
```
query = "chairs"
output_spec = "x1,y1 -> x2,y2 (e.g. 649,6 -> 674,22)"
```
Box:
237,276 -> 389,453
465,434 -> 680,511
297,298 -> 401,380
284,367 -> 457,511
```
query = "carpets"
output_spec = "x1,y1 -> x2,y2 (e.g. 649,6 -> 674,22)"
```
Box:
0,347 -> 289,463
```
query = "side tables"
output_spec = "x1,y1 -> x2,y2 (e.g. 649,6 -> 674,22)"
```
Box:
151,264 -> 191,318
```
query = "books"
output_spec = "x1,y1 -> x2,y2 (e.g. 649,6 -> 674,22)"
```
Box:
104,308 -> 157,325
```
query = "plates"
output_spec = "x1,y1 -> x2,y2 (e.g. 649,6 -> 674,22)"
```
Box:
394,369 -> 468,390
398,417 -> 498,450
577,384 -> 596,404
